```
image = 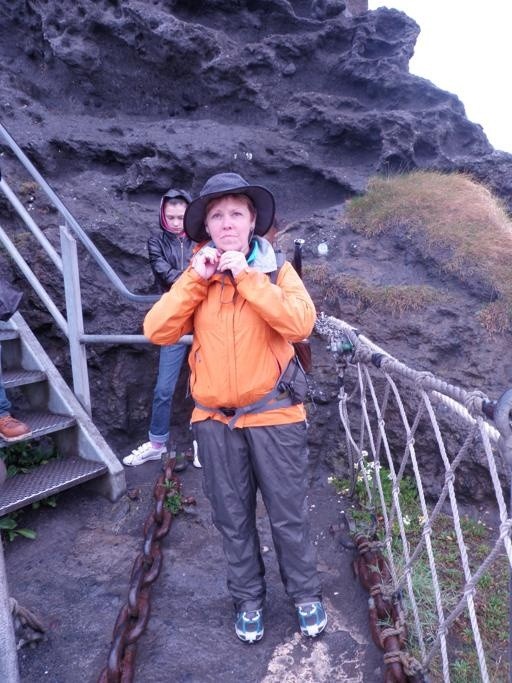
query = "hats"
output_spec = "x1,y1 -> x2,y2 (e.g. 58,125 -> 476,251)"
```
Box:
183,172 -> 277,243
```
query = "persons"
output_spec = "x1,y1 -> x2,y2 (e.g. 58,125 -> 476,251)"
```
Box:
119,187 -> 203,470
140,169 -> 331,646
1,251 -> 33,442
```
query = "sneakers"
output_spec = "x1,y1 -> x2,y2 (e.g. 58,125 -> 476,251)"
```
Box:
295,602 -> 329,638
0,417 -> 33,443
235,609 -> 264,644
123,442 -> 167,466
192,439 -> 202,468
